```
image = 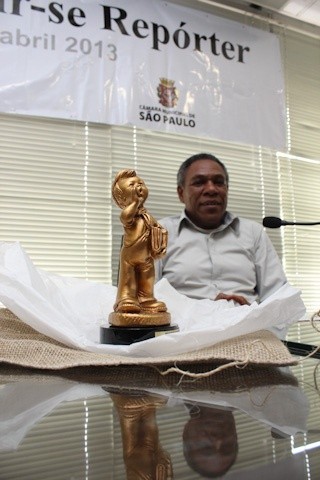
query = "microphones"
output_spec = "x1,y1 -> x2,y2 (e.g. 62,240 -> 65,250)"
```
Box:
262,216 -> 320,230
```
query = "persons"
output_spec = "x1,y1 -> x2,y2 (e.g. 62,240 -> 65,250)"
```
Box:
111,394 -> 174,480
181,401 -> 238,477
154,152 -> 291,338
110,169 -> 169,314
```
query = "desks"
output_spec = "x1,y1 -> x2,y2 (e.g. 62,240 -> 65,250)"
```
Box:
0,319 -> 319,480
